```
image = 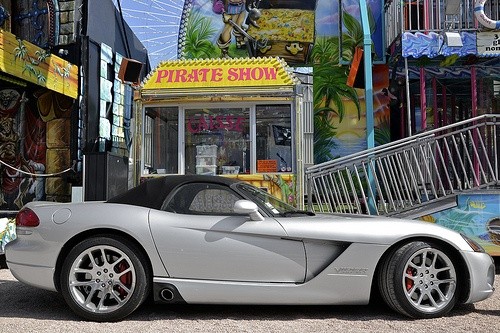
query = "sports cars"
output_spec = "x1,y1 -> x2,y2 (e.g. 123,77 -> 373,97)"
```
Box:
4,175 -> 496,322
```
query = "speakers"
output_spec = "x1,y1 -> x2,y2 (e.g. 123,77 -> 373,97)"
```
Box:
81,151 -> 129,204
118,57 -> 143,82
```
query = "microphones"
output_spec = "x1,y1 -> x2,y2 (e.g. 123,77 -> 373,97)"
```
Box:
276,153 -> 287,165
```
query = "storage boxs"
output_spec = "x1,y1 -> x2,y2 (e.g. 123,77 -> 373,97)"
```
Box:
221,166 -> 240,175
195,144 -> 218,155
195,155 -> 218,165
195,164 -> 218,176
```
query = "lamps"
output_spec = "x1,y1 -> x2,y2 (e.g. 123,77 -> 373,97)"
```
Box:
436,30 -> 464,54
